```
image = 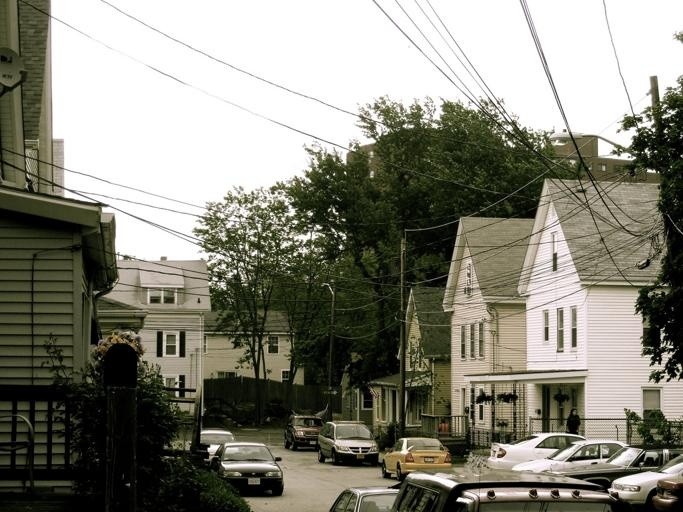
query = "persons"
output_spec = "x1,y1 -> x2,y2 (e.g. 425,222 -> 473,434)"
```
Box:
438,419 -> 448,437
566,408 -> 581,434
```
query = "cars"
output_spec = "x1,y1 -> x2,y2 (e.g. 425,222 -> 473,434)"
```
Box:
378,435 -> 454,482
192,426 -> 241,466
207,440 -> 287,499
329,484 -> 403,511
483,431 -> 683,512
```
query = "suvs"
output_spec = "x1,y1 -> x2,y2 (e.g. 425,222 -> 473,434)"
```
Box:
385,466 -> 623,510
281,411 -> 328,451
314,418 -> 381,468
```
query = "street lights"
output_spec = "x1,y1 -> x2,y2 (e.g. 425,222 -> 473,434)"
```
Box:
320,279 -> 338,420
549,130 -> 678,331
